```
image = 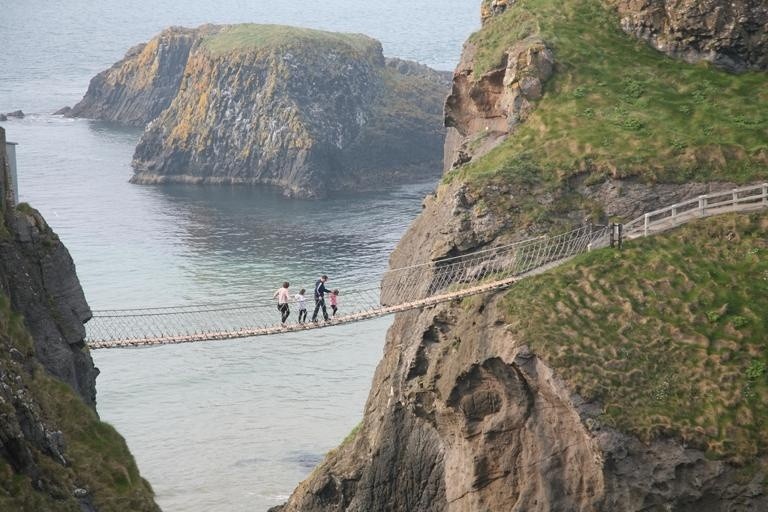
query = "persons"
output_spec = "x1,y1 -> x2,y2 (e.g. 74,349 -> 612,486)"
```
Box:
312,275 -> 332,322
330,289 -> 339,316
295,289 -> 307,324
273,282 -> 292,328
